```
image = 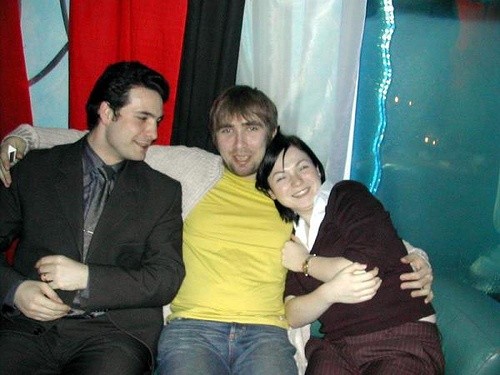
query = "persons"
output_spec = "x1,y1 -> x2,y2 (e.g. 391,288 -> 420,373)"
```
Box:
0,85 -> 435,375
0,60 -> 185,374
254,133 -> 448,375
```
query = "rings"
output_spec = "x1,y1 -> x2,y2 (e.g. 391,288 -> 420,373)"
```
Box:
42,273 -> 48,284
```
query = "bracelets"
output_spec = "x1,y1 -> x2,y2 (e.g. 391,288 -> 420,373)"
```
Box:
300,254 -> 315,278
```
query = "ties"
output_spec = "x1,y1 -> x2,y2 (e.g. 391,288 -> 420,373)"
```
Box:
82,165 -> 116,264
